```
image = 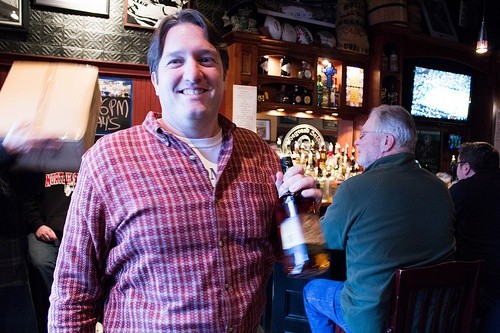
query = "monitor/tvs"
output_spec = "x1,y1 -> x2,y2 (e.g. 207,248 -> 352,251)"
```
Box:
406,61 -> 477,126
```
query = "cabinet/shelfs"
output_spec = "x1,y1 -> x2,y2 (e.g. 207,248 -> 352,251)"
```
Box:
222,24 -> 488,180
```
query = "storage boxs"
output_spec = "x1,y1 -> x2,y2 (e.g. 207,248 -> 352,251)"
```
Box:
0,60 -> 103,172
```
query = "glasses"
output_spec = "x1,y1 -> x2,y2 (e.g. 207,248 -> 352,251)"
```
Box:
359,130 -> 388,139
452,161 -> 464,171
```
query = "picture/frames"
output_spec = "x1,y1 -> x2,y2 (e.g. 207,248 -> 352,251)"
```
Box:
33,0 -> 111,18
124,0 -> 190,31
0,0 -> 30,36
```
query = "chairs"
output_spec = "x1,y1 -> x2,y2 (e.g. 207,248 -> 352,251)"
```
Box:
334,258 -> 484,333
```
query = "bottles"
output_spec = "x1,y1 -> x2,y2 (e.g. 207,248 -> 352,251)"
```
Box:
275,156 -> 330,278
449,136 -> 462,150
269,137 -> 363,202
258,54 -> 312,105
450,155 -> 458,181
333,78 -> 339,107
317,75 -> 323,107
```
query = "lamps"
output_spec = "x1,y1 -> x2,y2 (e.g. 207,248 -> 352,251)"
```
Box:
476,4 -> 488,54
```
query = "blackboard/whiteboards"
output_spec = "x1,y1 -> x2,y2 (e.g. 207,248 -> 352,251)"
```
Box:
95,95 -> 132,136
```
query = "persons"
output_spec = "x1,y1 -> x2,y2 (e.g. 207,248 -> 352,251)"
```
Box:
416,140 -> 433,173
302,104 -> 458,333
0,122 -> 81,293
49,7 -> 323,333
448,143 -> 500,323
